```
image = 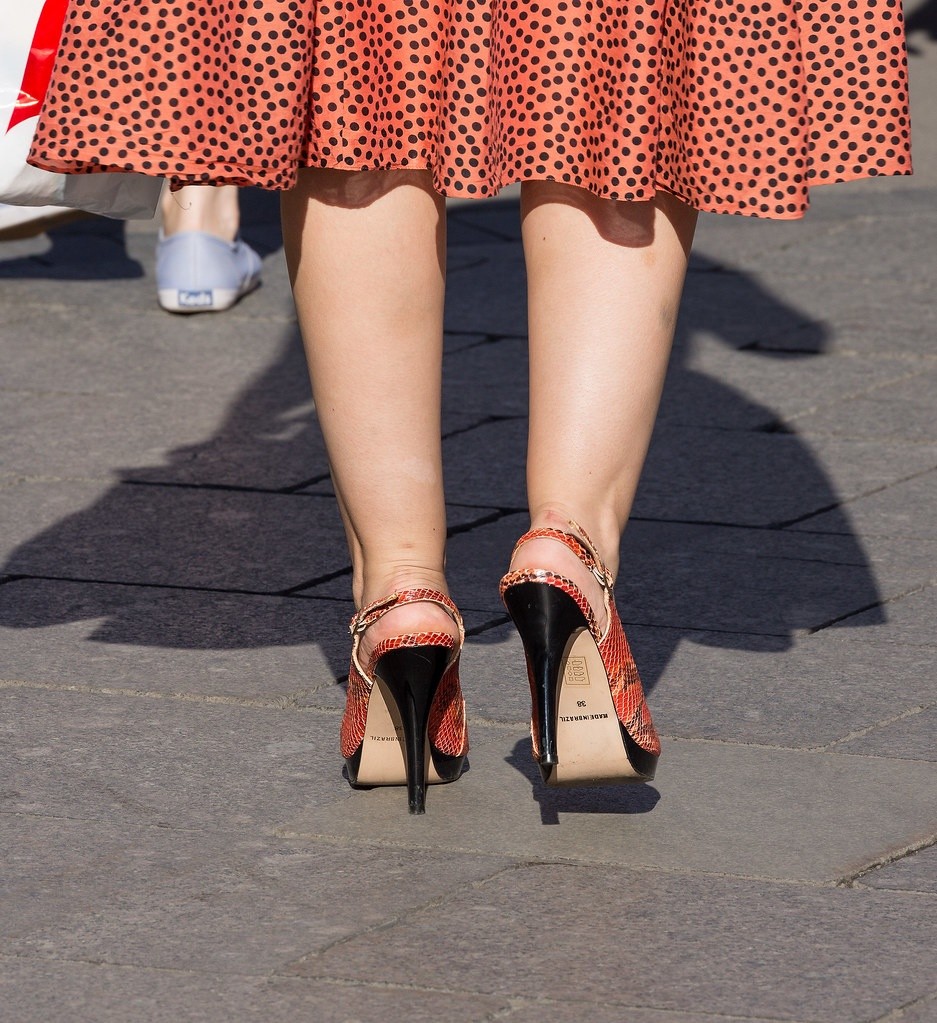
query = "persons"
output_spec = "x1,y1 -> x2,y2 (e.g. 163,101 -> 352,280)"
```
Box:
42,163 -> 263,316
27,0 -> 918,814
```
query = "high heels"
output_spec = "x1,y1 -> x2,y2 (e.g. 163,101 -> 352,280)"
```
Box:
499,519 -> 661,785
339,588 -> 469,814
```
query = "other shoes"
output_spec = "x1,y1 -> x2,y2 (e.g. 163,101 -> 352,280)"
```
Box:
157,228 -> 261,311
